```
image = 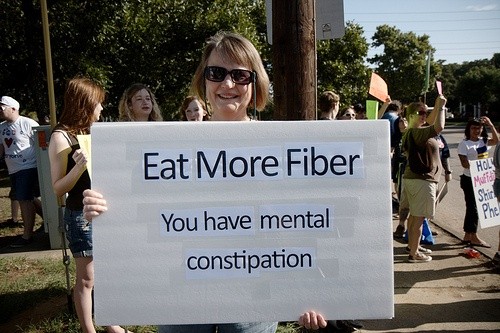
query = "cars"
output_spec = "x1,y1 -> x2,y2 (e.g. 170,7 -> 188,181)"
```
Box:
427,106 -> 455,122
457,111 -> 478,123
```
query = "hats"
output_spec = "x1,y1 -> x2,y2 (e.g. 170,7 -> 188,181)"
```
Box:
0,96 -> 19,110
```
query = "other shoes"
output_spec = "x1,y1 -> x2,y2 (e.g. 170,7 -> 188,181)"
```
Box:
407,253 -> 432,263
393,224 -> 405,238
406,246 -> 432,254
0,219 -> 18,228
491,252 -> 500,268
5,238 -> 32,252
34,223 -> 45,233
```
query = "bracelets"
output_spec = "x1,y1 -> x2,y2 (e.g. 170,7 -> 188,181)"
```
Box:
438,107 -> 445,111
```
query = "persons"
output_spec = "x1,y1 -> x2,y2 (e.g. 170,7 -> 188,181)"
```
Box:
83,31 -> 327,333
48,77 -> 135,333
0,96 -> 45,248
117,83 -> 163,122
318,89 -> 500,265
180,97 -> 209,122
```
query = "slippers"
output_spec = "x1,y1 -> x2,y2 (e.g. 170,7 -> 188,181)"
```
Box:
470,241 -> 491,248
463,239 -> 471,243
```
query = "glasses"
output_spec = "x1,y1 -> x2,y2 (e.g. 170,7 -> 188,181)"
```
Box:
0,106 -> 11,110
345,114 -> 355,117
415,110 -> 428,116
206,65 -> 256,86
